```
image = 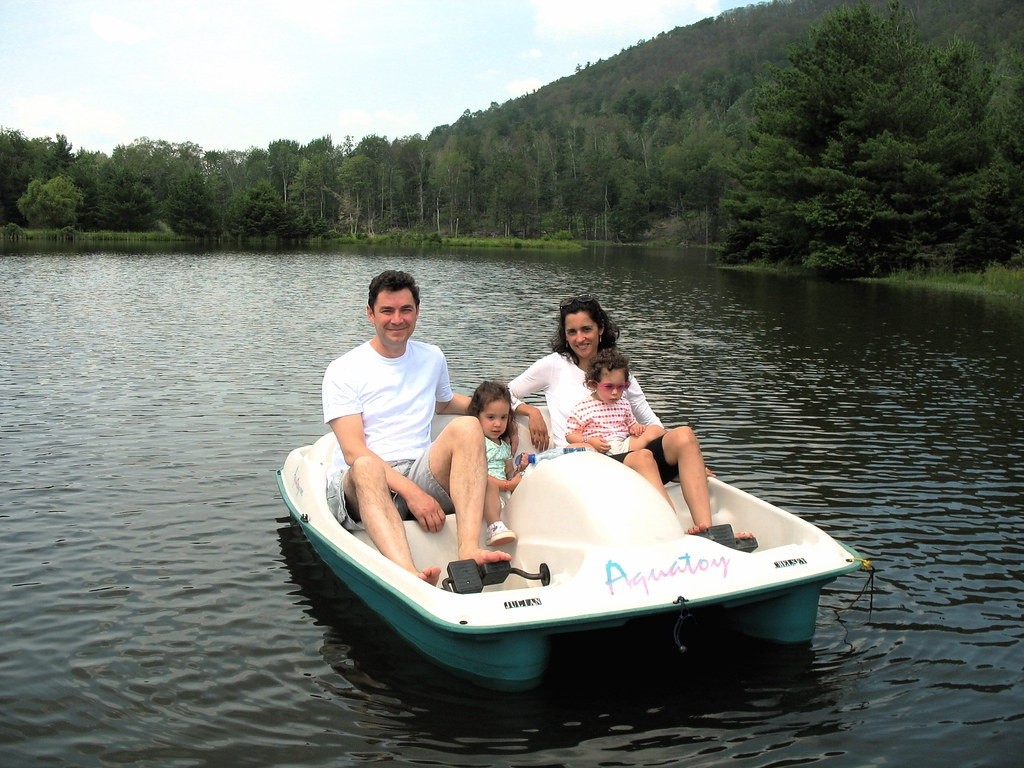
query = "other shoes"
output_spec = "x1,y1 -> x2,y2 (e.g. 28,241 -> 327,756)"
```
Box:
484,521 -> 516,546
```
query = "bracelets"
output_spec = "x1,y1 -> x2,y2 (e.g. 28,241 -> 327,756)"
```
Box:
583,434 -> 588,444
503,481 -> 509,491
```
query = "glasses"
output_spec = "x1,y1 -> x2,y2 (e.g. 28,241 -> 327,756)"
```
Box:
590,379 -> 631,391
559,294 -> 598,311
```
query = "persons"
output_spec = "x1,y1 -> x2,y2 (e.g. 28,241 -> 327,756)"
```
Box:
565,347 -> 688,484
510,294 -> 755,540
460,381 -> 530,547
323,268 -> 511,589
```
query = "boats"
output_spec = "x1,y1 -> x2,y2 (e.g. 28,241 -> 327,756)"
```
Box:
272,408 -> 862,690
